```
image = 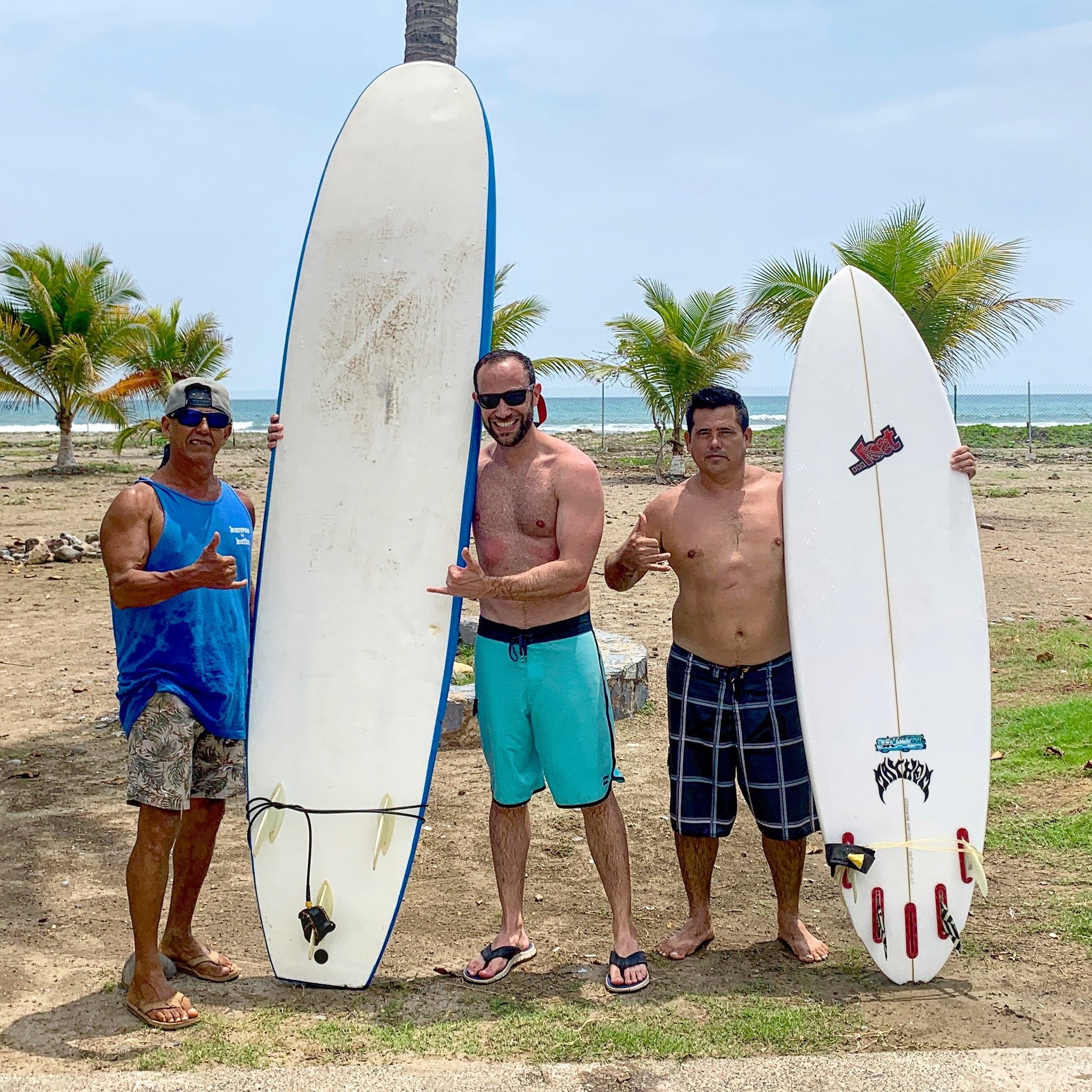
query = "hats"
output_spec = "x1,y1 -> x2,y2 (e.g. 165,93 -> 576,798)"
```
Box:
166,377 -> 231,417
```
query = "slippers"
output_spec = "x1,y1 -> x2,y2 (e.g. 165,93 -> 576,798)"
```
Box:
463,942 -> 537,985
176,948 -> 240,983
605,950 -> 649,993
127,991 -> 200,1031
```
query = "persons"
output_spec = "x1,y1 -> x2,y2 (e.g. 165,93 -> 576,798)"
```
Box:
99,380 -> 256,1030
269,348 -> 649,995
603,384 -> 976,962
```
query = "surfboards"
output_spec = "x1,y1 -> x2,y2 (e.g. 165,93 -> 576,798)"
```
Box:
243,57 -> 497,992
779,263 -> 994,987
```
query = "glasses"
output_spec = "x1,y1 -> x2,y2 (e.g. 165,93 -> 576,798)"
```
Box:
476,383 -> 535,410
167,409 -> 232,429
